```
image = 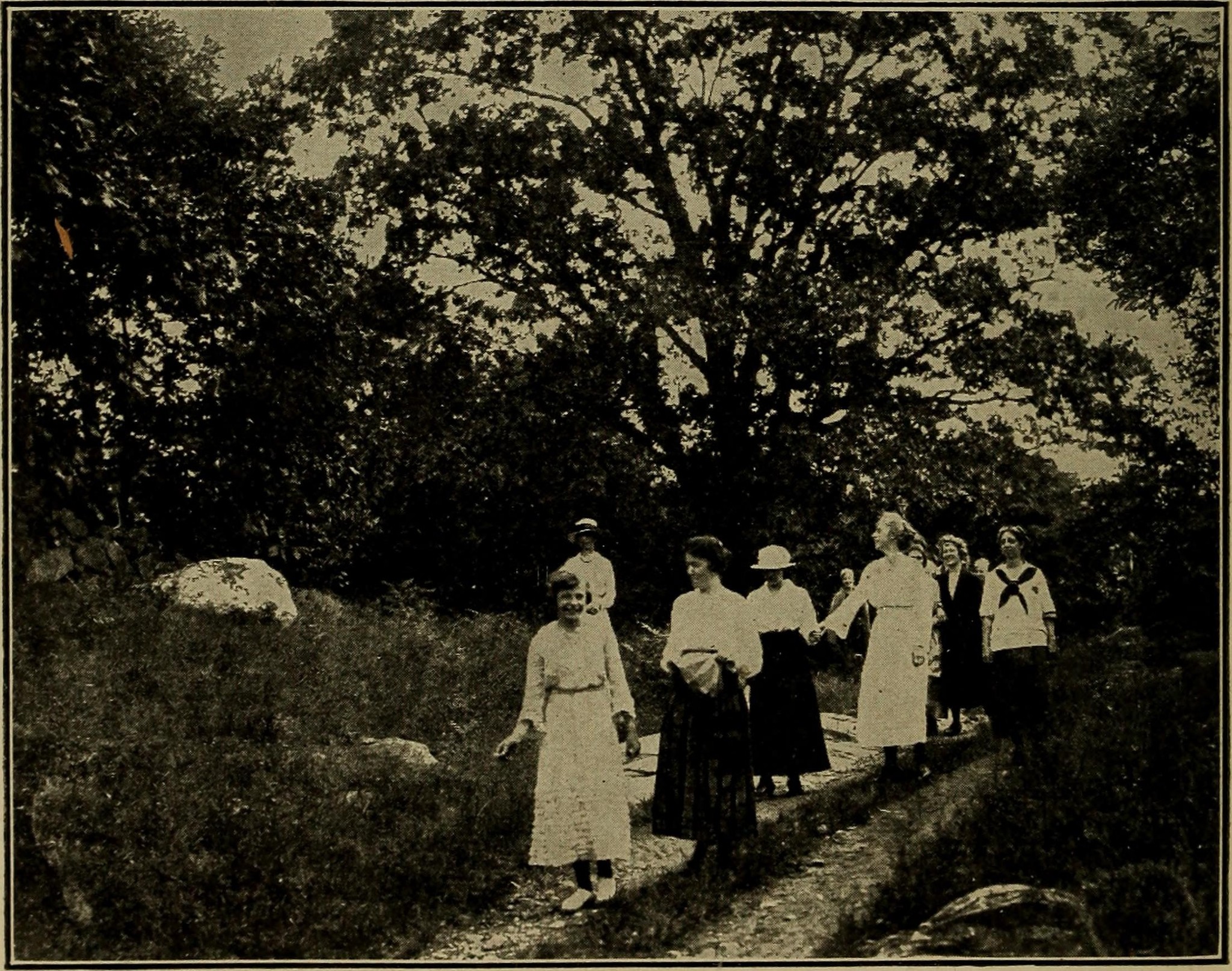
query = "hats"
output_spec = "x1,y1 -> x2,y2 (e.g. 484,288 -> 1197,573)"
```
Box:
750,544 -> 795,570
567,518 -> 603,544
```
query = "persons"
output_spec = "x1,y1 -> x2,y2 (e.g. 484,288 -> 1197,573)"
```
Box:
652,535 -> 764,875
747,545 -> 832,797
818,510 -> 1058,792
493,569 -> 643,912
560,518 -> 642,757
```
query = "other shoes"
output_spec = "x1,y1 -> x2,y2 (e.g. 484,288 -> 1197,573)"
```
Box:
787,779 -> 804,794
944,724 -> 960,736
755,781 -> 775,796
596,877 -> 616,903
876,762 -> 902,784
562,888 -> 592,911
914,755 -> 932,780
